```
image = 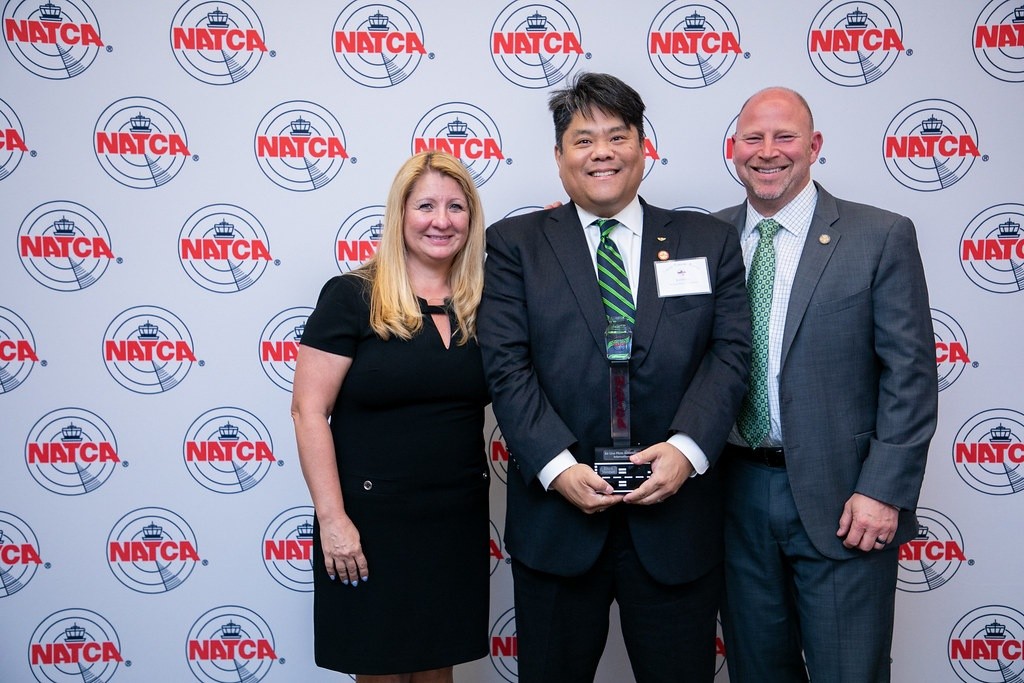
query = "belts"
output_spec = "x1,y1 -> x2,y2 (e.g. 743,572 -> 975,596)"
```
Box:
732,444 -> 785,466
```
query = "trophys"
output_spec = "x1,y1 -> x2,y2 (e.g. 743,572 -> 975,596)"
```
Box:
592,316 -> 653,493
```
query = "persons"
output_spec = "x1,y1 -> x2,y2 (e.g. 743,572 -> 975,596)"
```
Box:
290,151 -> 492,683
475,71 -> 753,683
545,86 -> 938,683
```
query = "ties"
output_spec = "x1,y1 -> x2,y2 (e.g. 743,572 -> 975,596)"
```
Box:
734,218 -> 782,450
591,219 -> 637,362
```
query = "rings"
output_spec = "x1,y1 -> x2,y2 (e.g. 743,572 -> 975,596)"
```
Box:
876,538 -> 886,544
656,499 -> 663,502
